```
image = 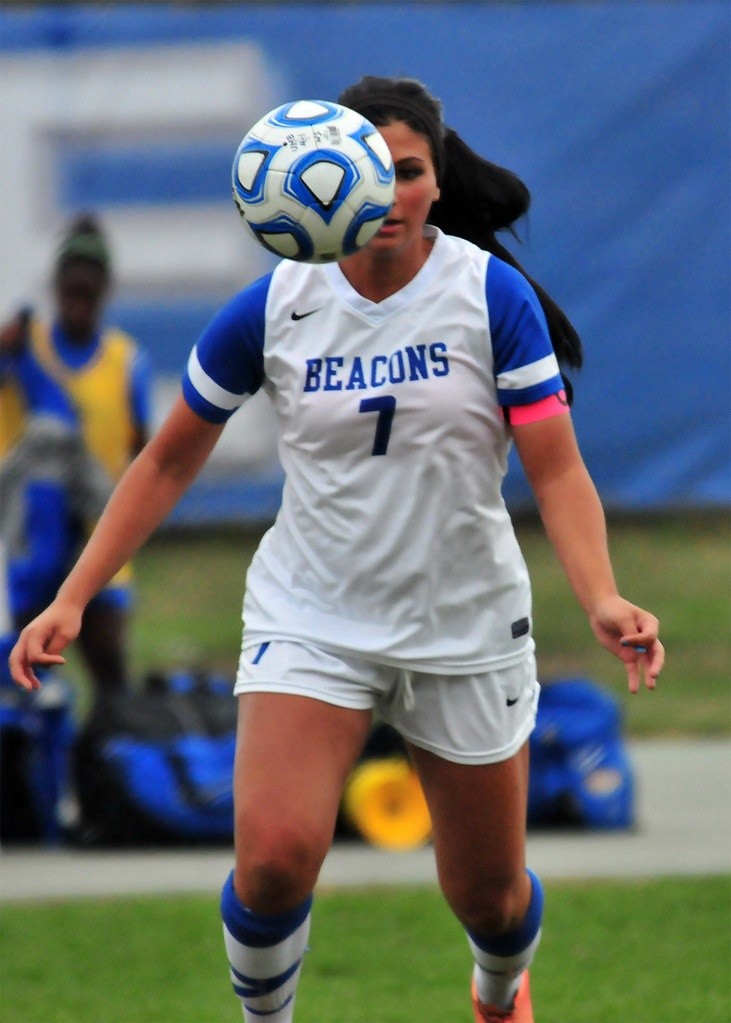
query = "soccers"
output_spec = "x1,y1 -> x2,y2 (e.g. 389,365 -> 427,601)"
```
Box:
230,98 -> 396,266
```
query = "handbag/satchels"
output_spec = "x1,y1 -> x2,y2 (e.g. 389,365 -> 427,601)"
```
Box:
524,678 -> 633,830
78,677 -> 242,841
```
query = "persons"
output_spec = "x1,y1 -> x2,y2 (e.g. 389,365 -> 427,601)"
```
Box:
7,76 -> 666,1022
0,208 -> 151,729
